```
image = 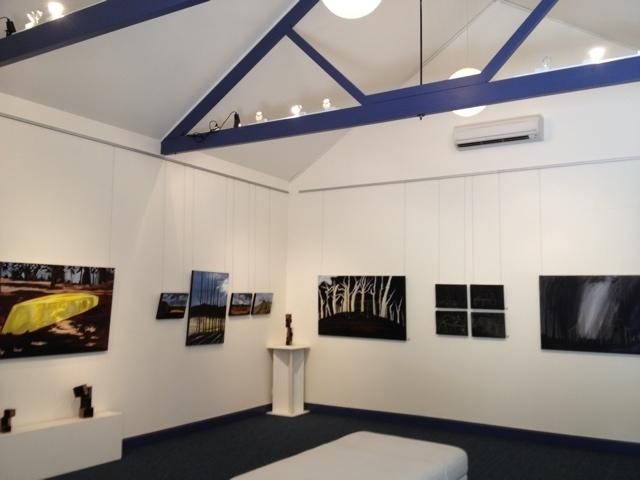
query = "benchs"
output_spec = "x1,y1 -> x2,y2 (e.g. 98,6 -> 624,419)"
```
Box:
230,431 -> 468,480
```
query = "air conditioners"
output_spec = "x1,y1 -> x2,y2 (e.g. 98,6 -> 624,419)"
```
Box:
453,115 -> 544,152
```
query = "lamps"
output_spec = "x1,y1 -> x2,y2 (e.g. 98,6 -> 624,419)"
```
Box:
448,0 -> 488,117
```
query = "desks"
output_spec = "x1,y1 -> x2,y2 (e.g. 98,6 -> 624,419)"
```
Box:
267,345 -> 310,417
